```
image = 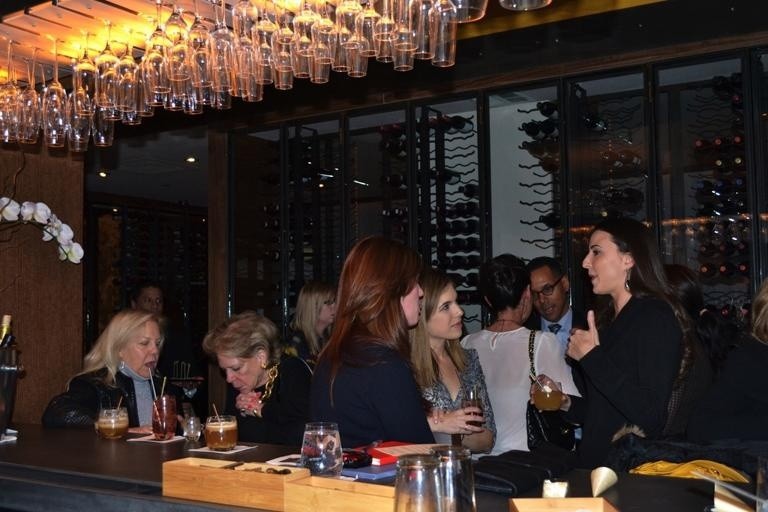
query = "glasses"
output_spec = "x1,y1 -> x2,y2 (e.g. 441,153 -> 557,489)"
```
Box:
531,274 -> 562,301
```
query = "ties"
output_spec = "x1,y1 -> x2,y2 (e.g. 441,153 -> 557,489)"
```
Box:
548,324 -> 561,334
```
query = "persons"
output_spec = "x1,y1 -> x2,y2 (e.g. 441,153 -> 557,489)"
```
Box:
306,236 -> 587,459
41,281 -> 338,445
530,217 -> 767,452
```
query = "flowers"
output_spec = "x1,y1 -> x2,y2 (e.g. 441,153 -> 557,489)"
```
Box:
0,143 -> 83,264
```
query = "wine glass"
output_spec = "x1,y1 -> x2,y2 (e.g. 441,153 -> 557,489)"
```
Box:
1,0 -> 456,153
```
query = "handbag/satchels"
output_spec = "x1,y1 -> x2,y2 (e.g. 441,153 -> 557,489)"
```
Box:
456,449 -> 562,497
526,395 -> 587,452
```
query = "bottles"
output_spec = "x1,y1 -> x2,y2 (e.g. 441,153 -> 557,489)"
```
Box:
102,209 -> 213,322
521,98 -> 767,322
253,114 -> 490,308
0,334 -> 15,351
0,313 -> 13,343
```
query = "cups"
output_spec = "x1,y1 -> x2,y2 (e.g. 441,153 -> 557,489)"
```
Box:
153,395 -> 178,441
497,0 -> 552,12
440,0 -> 488,23
392,453 -> 442,512
181,415 -> 201,444
430,445 -> 477,512
94,405 -> 130,440
299,420 -> 344,478
532,379 -> 563,413
205,415 -> 240,450
460,384 -> 483,429
